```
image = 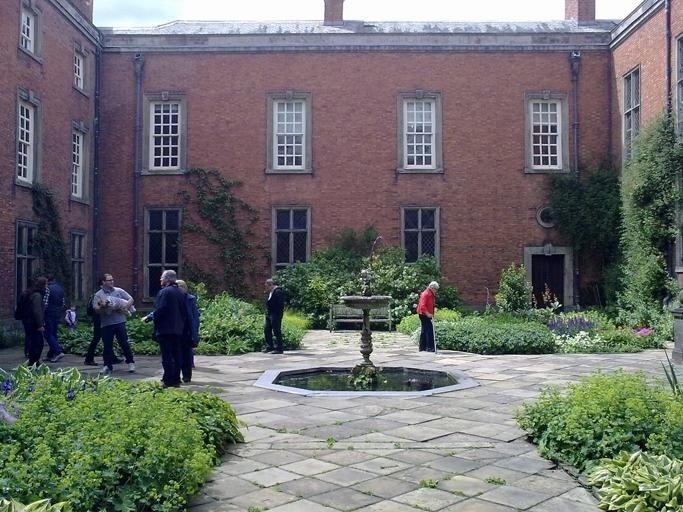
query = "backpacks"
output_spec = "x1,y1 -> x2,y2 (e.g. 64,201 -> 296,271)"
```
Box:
86,293 -> 96,317
13,288 -> 35,321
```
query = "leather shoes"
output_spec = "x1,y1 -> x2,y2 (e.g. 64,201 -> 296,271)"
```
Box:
83,361 -> 97,366
271,349 -> 282,354
183,376 -> 191,383
260,349 -> 273,353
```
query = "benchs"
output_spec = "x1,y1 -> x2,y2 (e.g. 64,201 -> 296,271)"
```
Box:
328,303 -> 392,332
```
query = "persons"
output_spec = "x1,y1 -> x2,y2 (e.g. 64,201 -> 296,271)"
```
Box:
139,279 -> 201,368
14,273 -> 136,375
261,278 -> 284,354
415,280 -> 440,354
150,269 -> 191,388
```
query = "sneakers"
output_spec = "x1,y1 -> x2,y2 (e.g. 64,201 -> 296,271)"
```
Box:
128,363 -> 134,372
50,353 -> 64,363
99,366 -> 112,376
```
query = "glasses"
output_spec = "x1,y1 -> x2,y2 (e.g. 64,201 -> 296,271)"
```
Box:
104,279 -> 114,282
